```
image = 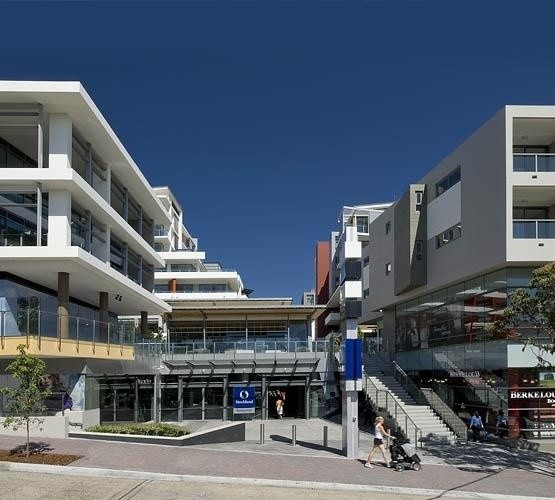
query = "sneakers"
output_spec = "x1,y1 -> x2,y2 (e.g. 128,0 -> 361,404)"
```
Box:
364,462 -> 373,468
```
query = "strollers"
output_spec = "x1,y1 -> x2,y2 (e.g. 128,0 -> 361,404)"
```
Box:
389,438 -> 421,472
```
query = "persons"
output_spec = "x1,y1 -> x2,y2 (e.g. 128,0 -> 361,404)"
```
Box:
365,416 -> 397,468
514,411 -> 528,440
497,410 -> 507,437
470,410 -> 484,442
275,395 -> 285,420
39,372 -> 72,413
